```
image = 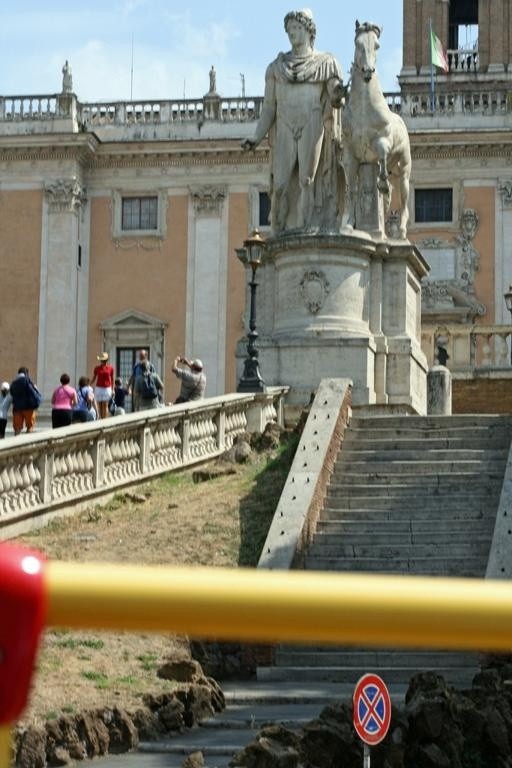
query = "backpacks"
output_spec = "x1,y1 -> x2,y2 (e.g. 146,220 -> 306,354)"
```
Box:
140,373 -> 158,399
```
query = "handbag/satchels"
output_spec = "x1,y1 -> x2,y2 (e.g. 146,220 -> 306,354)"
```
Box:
86,406 -> 96,422
174,396 -> 185,404
108,399 -> 115,415
26,379 -> 41,407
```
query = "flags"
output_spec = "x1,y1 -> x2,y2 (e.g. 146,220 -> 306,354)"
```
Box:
432,29 -> 449,73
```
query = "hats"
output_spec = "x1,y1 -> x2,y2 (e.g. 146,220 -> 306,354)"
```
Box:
190,359 -> 203,369
0,382 -> 9,391
96,351 -> 108,360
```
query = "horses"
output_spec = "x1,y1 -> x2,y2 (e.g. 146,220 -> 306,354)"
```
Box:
339,19 -> 412,240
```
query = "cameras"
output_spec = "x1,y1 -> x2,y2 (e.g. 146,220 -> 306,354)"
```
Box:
180,359 -> 185,363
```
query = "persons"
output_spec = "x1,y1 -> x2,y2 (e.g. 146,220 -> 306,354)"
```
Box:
241,10 -> 348,228
209,65 -> 217,93
0,348 -> 206,439
62,59 -> 72,92
